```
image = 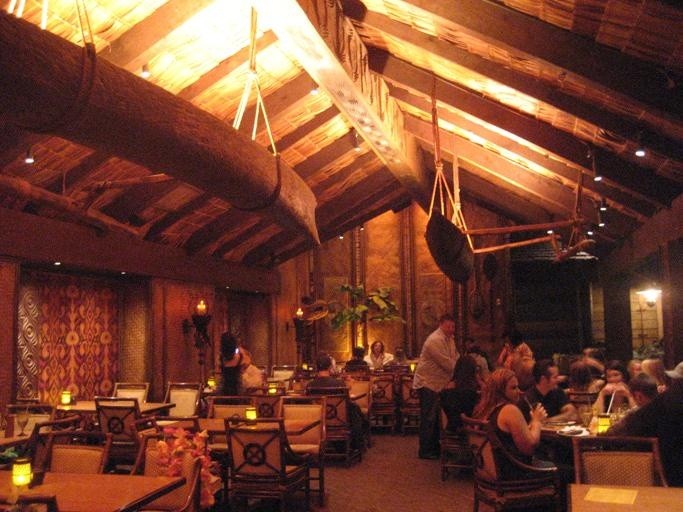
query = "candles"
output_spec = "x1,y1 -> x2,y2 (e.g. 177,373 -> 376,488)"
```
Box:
295,307 -> 304,318
196,300 -> 206,316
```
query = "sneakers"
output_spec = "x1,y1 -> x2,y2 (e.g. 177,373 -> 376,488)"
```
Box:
418,447 -> 440,460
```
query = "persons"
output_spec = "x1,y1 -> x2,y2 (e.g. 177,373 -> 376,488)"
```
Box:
411,313 -> 460,459
368,340 -> 387,369
382,347 -> 410,372
240,351 -> 266,393
452,333 -> 683,512
217,331 -> 251,399
307,353 -> 370,454
345,346 -> 370,376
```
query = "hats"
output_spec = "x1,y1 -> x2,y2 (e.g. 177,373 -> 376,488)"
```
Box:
664,360 -> 682,380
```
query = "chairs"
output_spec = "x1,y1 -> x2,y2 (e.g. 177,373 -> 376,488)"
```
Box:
0,363 -> 396,512
401,369 -> 683,511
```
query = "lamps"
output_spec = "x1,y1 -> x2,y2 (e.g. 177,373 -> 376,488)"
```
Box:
585,198 -> 610,237
635,138 -> 645,158
592,159 -> 602,182
635,283 -> 661,307
